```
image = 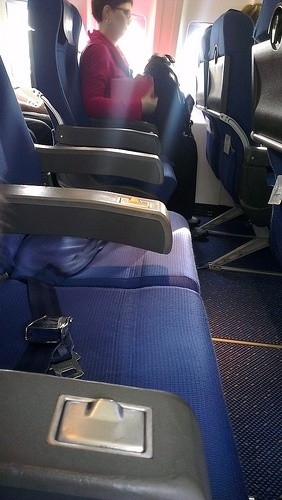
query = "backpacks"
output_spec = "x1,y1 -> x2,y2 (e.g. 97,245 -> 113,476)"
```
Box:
144,54 -> 198,173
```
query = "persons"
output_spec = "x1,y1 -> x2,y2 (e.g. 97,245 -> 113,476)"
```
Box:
79,0 -> 208,240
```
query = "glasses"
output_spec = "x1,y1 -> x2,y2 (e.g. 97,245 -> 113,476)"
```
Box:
112,7 -> 131,18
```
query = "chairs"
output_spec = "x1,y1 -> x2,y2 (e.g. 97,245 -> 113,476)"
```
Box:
0,0 -> 282,500
